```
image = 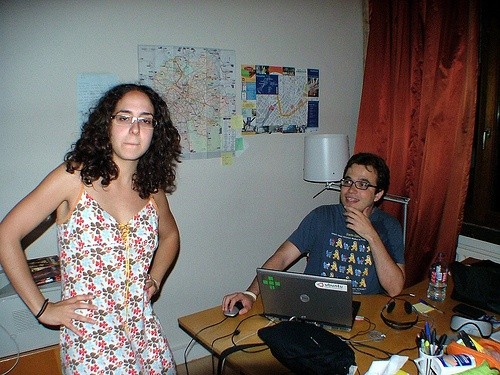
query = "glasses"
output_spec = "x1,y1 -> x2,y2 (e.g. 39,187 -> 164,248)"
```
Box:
340,178 -> 378,190
110,112 -> 159,129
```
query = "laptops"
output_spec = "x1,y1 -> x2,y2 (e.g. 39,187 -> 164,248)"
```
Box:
255,268 -> 361,328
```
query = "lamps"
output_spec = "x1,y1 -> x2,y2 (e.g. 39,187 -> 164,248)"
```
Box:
301,132 -> 411,252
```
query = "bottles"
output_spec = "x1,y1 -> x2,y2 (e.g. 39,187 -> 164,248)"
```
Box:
427,252 -> 448,302
431,354 -> 476,375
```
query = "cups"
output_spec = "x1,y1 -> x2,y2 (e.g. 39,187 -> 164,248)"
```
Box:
414,344 -> 443,375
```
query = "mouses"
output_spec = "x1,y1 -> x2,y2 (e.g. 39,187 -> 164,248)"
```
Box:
224,300 -> 243,318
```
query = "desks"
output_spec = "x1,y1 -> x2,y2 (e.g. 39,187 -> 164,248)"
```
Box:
178,256 -> 500,375
0,344 -> 62,375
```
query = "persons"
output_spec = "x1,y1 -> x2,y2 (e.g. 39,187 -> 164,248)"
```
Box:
221,153 -> 406,315
0,83 -> 183,375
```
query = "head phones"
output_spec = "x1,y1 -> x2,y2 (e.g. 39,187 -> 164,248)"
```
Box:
380,296 -> 420,329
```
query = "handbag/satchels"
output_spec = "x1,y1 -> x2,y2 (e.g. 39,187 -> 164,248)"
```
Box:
218,321 -> 357,375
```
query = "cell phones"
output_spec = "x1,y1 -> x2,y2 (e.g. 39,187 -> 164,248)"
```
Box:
452,303 -> 487,321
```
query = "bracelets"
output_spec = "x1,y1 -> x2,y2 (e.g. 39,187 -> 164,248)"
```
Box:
152,278 -> 161,286
242,291 -> 257,302
36,299 -> 50,319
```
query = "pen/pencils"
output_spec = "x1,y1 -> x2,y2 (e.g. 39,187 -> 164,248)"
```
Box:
416,322 -> 447,355
418,298 -> 443,313
